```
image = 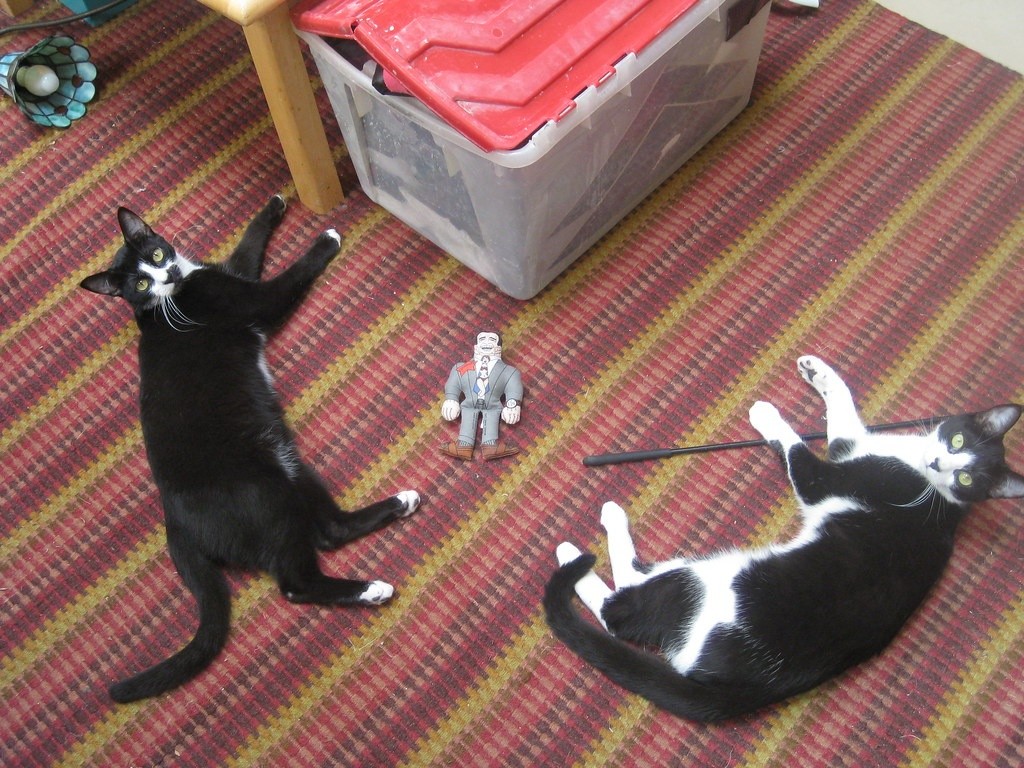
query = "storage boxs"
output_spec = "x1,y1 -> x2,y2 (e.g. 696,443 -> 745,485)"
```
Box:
290,0 -> 773,300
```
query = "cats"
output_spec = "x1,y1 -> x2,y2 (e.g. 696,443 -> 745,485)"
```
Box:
79,194 -> 420,703
543,355 -> 1024,723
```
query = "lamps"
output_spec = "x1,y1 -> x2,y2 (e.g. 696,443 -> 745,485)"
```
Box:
0,32 -> 98,129
